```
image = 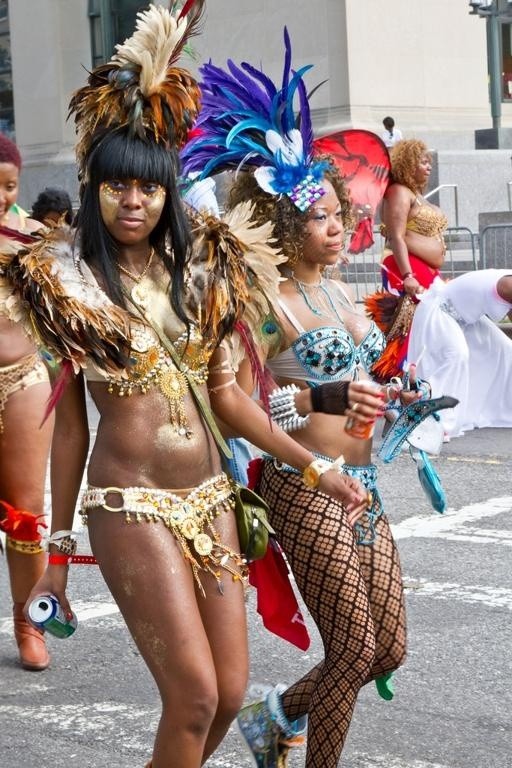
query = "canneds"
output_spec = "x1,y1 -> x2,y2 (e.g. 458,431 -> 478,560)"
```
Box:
27,594 -> 78,640
343,379 -> 381,440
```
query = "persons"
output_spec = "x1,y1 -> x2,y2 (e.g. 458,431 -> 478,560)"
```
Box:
379,139 -> 448,374
25,187 -> 75,225
406,268 -> 512,451
214,153 -> 425,768
0,119 -> 374,768
378,116 -> 401,148
0,134 -> 59,672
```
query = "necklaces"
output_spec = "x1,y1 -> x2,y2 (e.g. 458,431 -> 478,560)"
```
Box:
115,243 -> 156,309
289,272 -> 347,326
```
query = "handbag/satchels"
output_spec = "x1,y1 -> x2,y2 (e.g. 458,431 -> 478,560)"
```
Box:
230,481 -> 275,560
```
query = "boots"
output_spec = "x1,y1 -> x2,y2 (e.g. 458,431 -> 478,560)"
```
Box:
237,690 -> 307,767
13,601 -> 49,669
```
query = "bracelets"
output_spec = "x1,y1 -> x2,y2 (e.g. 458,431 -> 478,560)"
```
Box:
302,457 -> 344,489
401,272 -> 413,280
386,383 -> 393,401
266,384 -> 310,432
46,532 -> 77,553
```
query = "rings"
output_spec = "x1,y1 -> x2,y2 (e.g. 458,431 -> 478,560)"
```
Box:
366,490 -> 372,506
350,398 -> 360,412
